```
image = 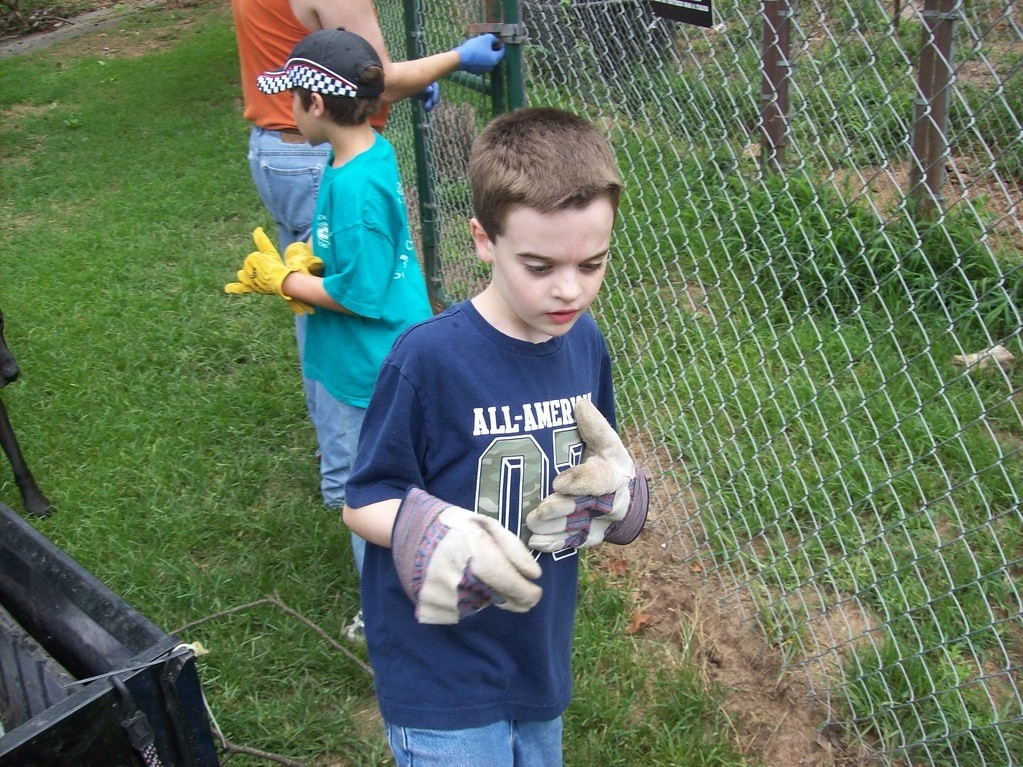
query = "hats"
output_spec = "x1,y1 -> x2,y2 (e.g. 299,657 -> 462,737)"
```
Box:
255,25 -> 383,98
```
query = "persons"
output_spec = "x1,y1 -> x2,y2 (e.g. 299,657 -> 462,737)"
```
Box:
224,27 -> 434,644
231,0 -> 506,260
342,107 -> 650,767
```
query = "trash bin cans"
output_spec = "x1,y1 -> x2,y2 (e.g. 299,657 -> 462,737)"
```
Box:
0,499 -> 222,767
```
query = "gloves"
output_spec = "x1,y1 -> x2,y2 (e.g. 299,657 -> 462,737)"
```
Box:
526,400 -> 650,552
281,241 -> 323,318
225,226 -> 299,300
412,80 -> 440,112
392,485 -> 542,625
456,34 -> 507,76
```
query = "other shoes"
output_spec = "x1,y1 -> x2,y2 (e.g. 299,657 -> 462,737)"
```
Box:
341,607 -> 366,642
